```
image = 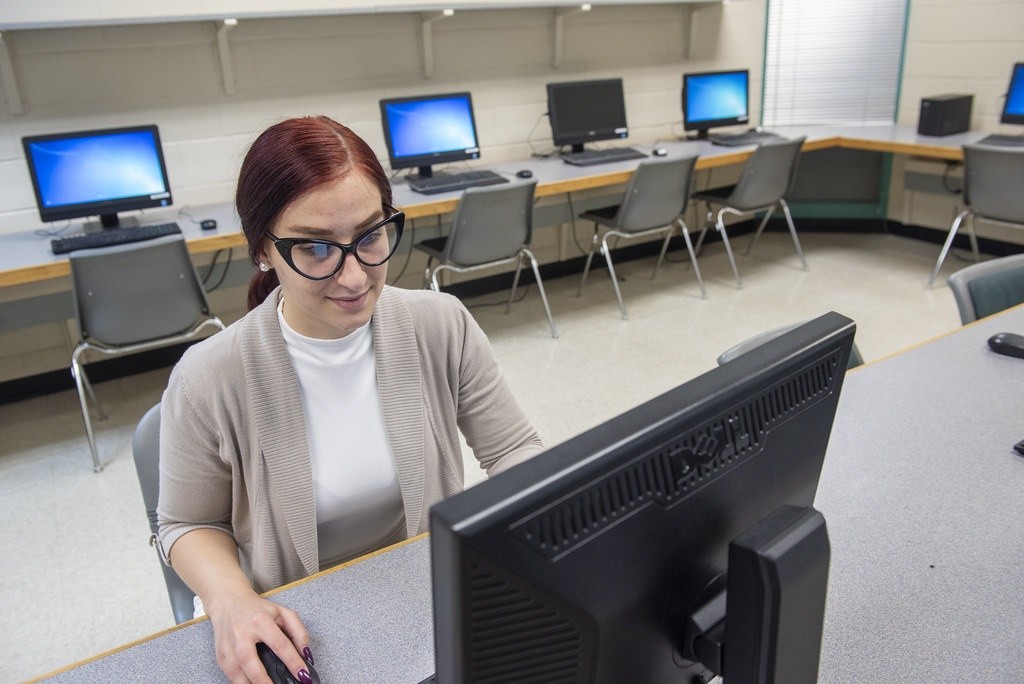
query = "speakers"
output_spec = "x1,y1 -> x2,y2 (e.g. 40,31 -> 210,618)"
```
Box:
919,95 -> 972,136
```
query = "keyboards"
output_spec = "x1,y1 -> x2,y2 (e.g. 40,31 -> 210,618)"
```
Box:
51,224 -> 177,255
408,170 -> 510,196
563,148 -> 648,166
417,673 -> 437,684
982,134 -> 1024,147
712,132 -> 778,147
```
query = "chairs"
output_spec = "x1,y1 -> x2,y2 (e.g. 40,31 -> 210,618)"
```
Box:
62,236 -> 224,473
413,178 -> 559,340
683,134 -> 807,288
575,152 -> 707,319
129,404 -> 205,624
718,321 -> 864,383
948,251 -> 1024,325
927,143 -> 1024,290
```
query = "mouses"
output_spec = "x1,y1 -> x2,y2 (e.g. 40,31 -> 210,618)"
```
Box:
987,333 -> 1024,359
516,170 -> 532,178
201,220 -> 216,230
254,642 -> 321,684
652,148 -> 667,156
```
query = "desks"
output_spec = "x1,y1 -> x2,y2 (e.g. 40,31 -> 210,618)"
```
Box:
0,125 -> 1024,684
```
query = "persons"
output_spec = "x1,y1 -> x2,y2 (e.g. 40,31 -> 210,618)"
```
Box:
148,116 -> 546,683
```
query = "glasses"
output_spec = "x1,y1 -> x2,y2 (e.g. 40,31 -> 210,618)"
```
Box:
264,203 -> 406,280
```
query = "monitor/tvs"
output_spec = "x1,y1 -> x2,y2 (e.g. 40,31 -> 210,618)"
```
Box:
379,93 -> 480,180
680,70 -> 749,140
547,78 -> 627,154
429,310 -> 857,684
21,125 -> 172,237
1001,61 -> 1024,125
